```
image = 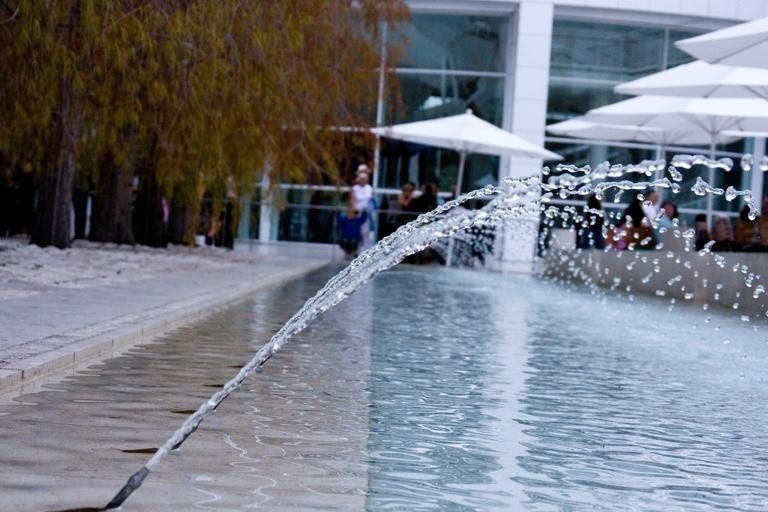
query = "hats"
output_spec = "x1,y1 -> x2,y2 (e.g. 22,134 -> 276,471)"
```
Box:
355,164 -> 372,176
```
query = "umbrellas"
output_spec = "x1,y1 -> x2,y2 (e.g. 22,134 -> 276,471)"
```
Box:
674,15 -> 766,72
541,114 -> 745,191
371,105 -> 566,268
612,59 -> 767,99
586,96 -> 766,233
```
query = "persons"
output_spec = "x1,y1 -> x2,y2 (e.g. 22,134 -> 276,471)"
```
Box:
335,163 -> 375,264
396,182 -> 456,228
579,188 -> 768,253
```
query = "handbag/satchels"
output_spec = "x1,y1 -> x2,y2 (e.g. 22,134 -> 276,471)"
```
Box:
337,215 -> 362,252
